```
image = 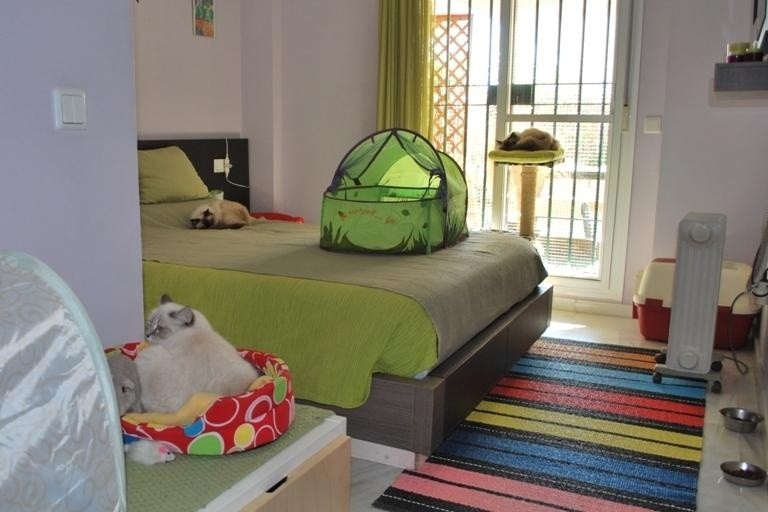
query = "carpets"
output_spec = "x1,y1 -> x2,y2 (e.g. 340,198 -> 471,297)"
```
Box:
369,335 -> 708,511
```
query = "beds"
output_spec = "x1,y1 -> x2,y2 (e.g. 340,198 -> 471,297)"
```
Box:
136,139 -> 556,470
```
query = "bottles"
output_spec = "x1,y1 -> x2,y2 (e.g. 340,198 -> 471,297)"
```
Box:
728,48 -> 763,63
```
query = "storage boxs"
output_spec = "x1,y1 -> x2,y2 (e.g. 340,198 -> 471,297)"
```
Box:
634,255 -> 760,350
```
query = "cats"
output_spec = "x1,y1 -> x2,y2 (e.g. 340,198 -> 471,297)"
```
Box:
189,199 -> 267,230
133,293 -> 266,415
495,127 -> 561,152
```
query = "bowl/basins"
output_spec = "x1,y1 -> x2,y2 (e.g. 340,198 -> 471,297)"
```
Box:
719,407 -> 764,432
719,461 -> 767,487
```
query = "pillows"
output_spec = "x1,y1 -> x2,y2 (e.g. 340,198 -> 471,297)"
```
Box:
137,145 -> 211,206
